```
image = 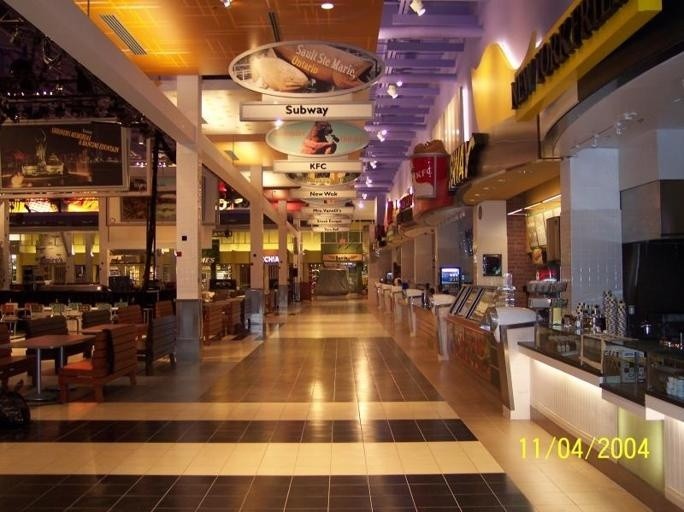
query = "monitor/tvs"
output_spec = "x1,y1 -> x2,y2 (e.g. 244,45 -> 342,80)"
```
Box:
440,266 -> 461,285
386,273 -> 393,284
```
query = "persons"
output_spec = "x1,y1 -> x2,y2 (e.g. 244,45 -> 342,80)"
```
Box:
386,270 -> 450,310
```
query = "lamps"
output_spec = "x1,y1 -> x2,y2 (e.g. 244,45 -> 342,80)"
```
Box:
409,0 -> 426,19
355,84 -> 402,213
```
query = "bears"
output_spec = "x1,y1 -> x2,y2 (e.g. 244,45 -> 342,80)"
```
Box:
299,120 -> 337,155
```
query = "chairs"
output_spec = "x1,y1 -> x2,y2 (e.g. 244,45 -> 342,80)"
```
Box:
0,288 -> 278,405
